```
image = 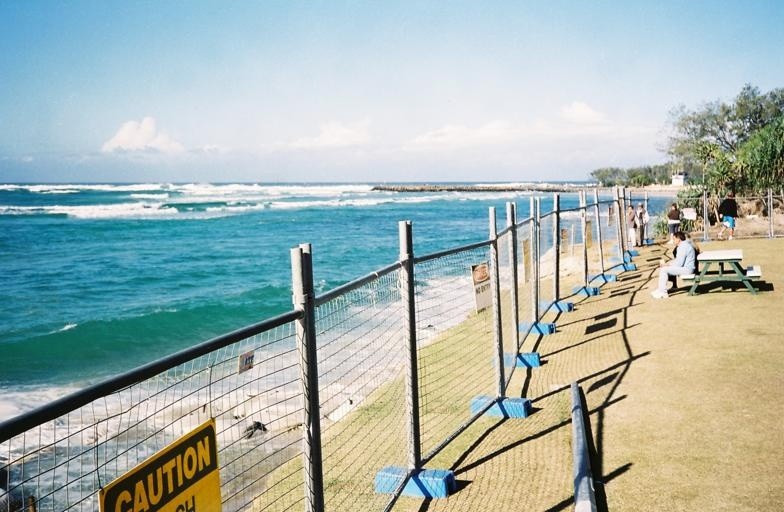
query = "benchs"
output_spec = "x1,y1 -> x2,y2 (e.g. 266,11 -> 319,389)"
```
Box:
680,264 -> 762,296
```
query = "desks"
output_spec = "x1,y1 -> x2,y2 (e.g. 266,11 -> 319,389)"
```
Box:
687,250 -> 757,295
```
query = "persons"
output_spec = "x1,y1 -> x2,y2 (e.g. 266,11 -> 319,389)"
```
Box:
666,203 -> 681,244
626,205 -> 638,247
717,191 -> 738,240
635,202 -> 647,246
649,232 -> 699,299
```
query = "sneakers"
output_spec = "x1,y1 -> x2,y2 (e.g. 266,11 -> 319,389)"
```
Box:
651,285 -> 679,299
631,233 -> 735,248
